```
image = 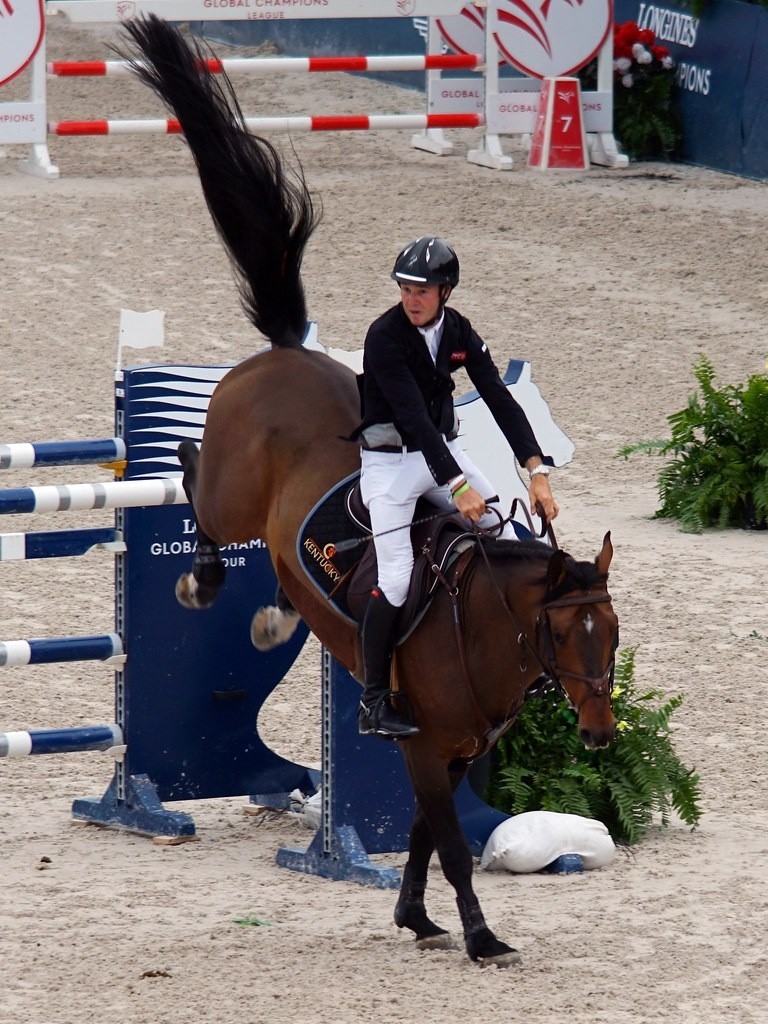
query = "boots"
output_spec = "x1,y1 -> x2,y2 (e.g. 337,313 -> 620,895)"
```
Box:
358,586 -> 420,738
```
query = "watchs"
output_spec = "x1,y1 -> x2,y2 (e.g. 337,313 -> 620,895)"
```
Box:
529,465 -> 550,478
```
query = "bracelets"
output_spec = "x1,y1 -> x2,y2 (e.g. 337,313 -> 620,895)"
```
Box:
446,474 -> 469,499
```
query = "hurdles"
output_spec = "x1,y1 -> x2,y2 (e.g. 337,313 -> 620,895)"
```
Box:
0,0 -> 630,177
1,322 -> 584,891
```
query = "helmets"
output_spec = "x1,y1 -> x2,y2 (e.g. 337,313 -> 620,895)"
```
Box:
389,237 -> 460,284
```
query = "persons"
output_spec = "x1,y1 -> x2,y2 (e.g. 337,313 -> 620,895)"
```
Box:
358,236 -> 559,735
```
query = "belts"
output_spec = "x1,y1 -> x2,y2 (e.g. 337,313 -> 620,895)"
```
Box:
362,444 -> 422,453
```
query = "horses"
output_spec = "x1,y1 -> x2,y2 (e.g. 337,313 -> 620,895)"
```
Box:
105,12 -> 623,967
